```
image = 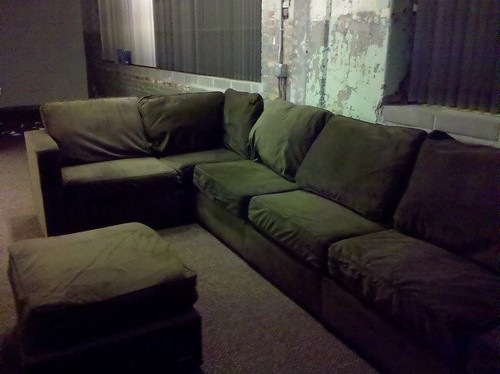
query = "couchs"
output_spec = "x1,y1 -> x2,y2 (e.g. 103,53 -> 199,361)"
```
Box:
194,98 -> 500,374
23,88 -> 264,237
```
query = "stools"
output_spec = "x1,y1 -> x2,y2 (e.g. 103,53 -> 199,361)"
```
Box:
5,222 -> 204,374
